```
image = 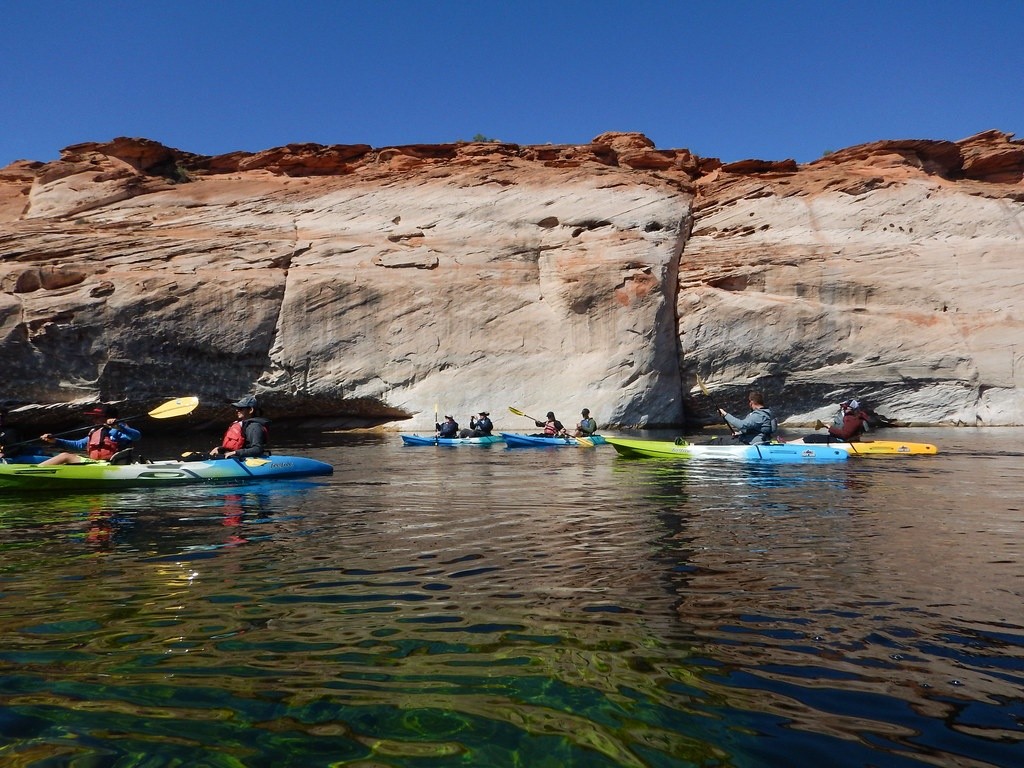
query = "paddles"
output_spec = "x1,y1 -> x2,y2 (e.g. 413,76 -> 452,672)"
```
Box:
814,419 -> 829,431
696,374 -> 735,434
507,405 -> 593,447
181,451 -> 272,467
433,399 -> 439,446
0,396 -> 200,451
589,434 -> 615,439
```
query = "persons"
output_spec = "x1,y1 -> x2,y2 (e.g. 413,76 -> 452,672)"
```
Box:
524,412 -> 566,438
570,409 -> 596,438
453,412 -> 493,438
777,400 -> 870,444
675,391 -> 777,445
38,408 -> 140,465
435,415 -> 458,438
178,396 -> 268,459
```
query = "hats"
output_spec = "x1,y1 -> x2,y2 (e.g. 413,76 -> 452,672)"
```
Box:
546,412 -> 554,418
840,400 -> 859,409
580,408 -> 589,414
230,393 -> 263,408
444,414 -> 454,419
82,403 -> 120,418
478,412 -> 490,417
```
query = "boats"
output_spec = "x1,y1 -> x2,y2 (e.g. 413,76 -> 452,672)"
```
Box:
759,440 -> 939,456
401,433 -> 506,446
498,432 -> 610,448
0,446 -> 333,493
604,436 -> 851,462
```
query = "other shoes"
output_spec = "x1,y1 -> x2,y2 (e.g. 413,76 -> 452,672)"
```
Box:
777,436 -> 786,444
675,437 -> 687,446
